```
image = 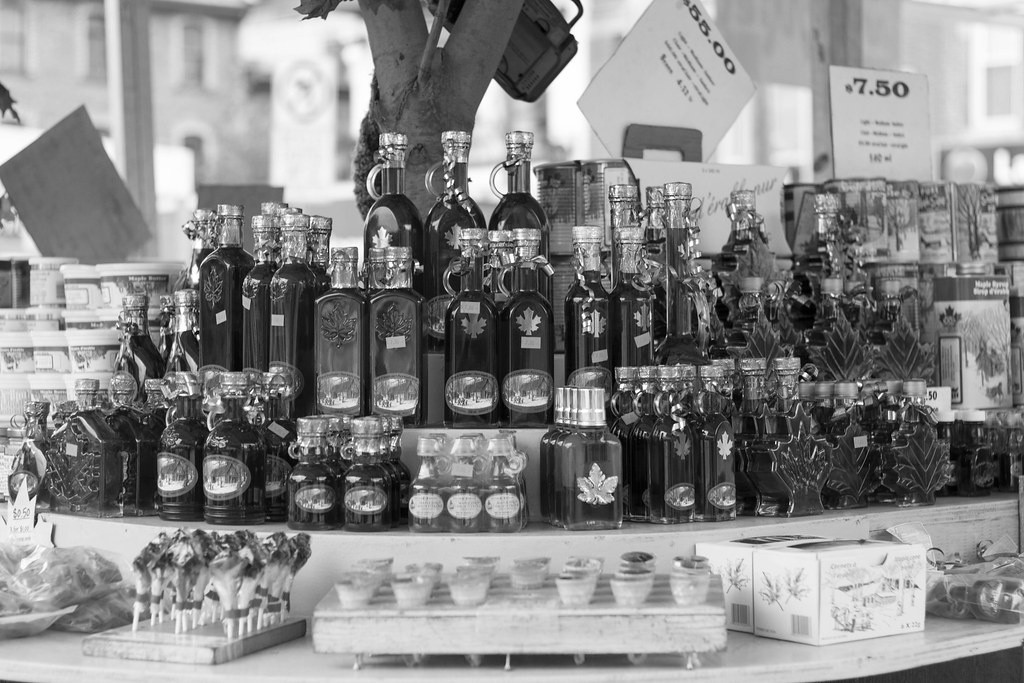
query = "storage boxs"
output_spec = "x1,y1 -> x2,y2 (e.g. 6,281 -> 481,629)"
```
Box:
823,178 -> 1024,411
752,532 -> 927,648
690,530 -> 821,635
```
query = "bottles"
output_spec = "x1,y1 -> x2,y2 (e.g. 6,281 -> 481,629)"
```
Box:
364,132 -> 424,292
409,431 -> 528,534
8,369 -> 296,526
290,416 -> 411,531
115,290 -> 199,400
445,228 -> 555,429
725,278 -> 935,381
939,409 -> 1023,498
718,190 -> 875,330
609,182 -> 718,366
315,247 -> 425,428
712,359 -> 834,517
183,202 -> 332,417
612,366 -> 737,525
800,381 -> 950,510
564,225 -> 655,387
540,387 -> 623,530
424,131 -> 488,353
488,132 -> 550,315
